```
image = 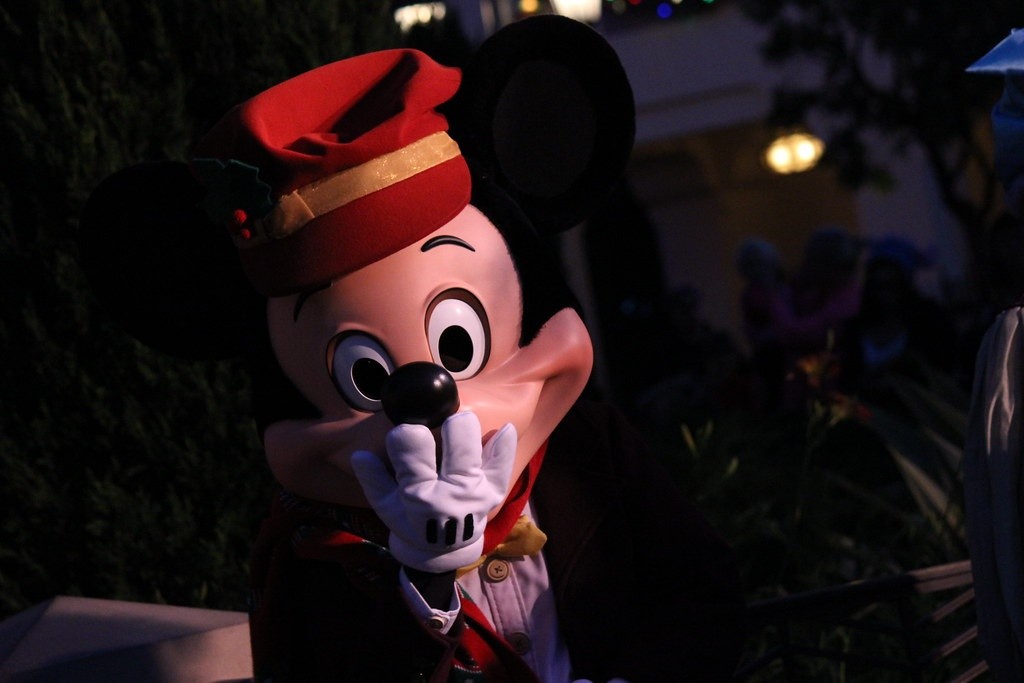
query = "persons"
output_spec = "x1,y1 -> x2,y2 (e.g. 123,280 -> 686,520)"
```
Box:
735,231 -> 874,412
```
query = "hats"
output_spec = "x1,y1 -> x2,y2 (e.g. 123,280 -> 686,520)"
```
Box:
460,15 -> 639,238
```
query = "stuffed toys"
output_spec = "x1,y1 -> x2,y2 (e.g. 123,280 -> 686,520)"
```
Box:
78,15 -> 743,683
